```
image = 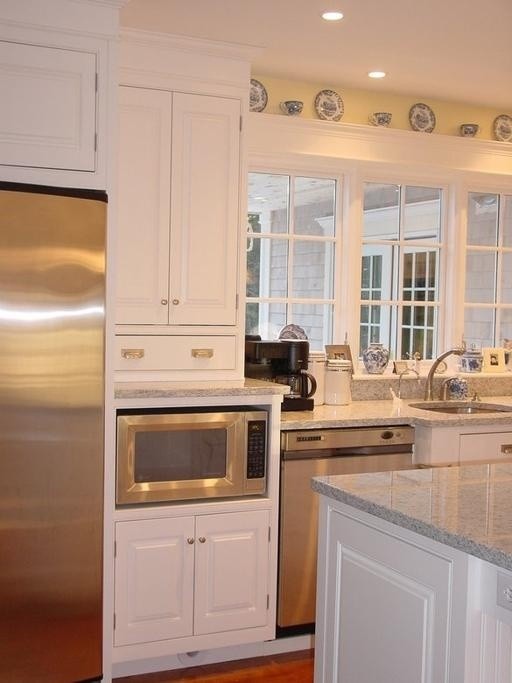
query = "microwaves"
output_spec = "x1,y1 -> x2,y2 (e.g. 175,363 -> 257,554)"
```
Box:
117,405 -> 268,505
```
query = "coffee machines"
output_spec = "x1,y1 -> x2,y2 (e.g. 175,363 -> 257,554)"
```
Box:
245,339 -> 317,412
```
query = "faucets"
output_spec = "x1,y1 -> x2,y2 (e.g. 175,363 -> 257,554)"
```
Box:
424,347 -> 466,400
398,369 -> 421,399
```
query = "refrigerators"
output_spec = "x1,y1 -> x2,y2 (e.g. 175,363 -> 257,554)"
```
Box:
0,182 -> 108,683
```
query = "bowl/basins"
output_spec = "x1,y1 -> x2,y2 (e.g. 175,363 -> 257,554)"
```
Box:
458,124 -> 479,137
279,100 -> 303,116
368,113 -> 392,126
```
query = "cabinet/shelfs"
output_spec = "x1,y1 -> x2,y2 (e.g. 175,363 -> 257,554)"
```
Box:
312,493 -> 512,682
112,84 -> 243,385
106,396 -> 277,665
0,39 -> 98,173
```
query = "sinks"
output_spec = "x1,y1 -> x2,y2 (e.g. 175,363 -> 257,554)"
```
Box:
408,400 -> 512,414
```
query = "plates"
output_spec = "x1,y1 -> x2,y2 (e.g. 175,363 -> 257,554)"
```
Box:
408,103 -> 436,132
494,115 -> 511,142
278,324 -> 308,339
314,89 -> 345,122
250,78 -> 268,112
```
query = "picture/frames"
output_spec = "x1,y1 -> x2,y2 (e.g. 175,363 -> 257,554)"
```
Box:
482,347 -> 506,373
326,345 -> 354,376
